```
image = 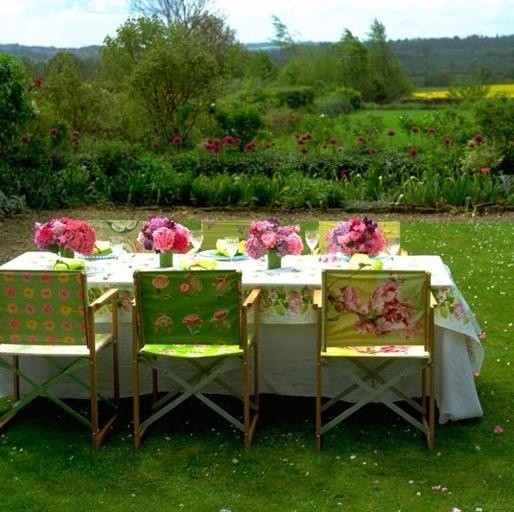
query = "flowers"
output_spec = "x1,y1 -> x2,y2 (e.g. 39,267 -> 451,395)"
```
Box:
428,282 -> 470,325
86,284 -> 136,315
325,276 -> 426,342
325,214 -> 386,257
241,216 -> 308,259
256,284 -> 311,319
134,216 -> 192,255
30,214 -> 95,254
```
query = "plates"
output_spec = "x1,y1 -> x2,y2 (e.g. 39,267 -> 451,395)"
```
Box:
40,263 -> 105,277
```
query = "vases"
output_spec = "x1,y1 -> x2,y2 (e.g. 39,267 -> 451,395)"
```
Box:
158,249 -> 173,267
60,246 -> 73,259
267,249 -> 282,270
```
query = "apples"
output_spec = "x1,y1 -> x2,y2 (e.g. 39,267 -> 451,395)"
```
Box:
373,259 -> 383,269
70,260 -> 84,270
55,263 -> 68,270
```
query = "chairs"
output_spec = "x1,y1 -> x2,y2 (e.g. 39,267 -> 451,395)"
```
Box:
84,218 -> 151,254
315,219 -> 402,256
128,266 -> 264,454
196,218 -> 252,254
309,267 -> 441,452
1,266 -> 122,448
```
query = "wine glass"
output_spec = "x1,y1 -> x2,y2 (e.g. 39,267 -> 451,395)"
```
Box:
225,236 -> 240,268
188,230 -> 207,260
386,233 -> 400,269
304,230 -> 320,265
109,236 -> 126,267
